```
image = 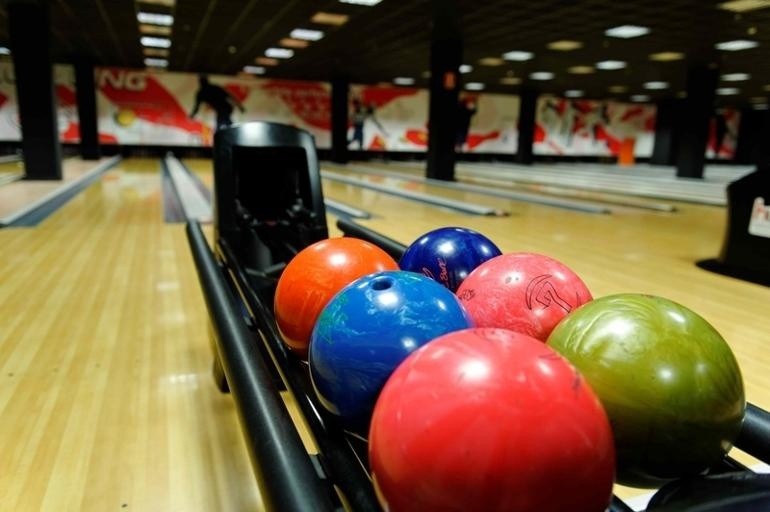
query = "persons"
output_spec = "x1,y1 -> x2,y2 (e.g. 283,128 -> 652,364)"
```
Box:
187,76 -> 246,130
348,98 -> 382,150
708,111 -> 736,158
